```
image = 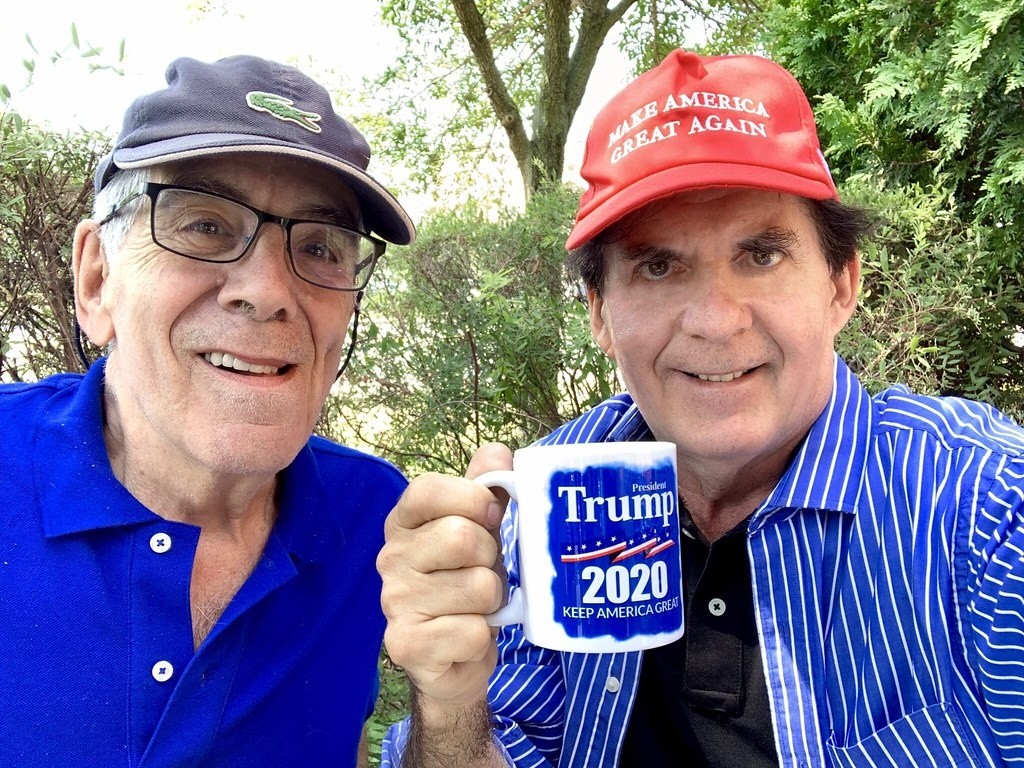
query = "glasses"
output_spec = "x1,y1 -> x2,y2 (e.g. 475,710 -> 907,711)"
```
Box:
95,181 -> 386,292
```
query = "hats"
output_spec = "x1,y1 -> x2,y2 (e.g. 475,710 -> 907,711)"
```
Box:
565,49 -> 840,251
95,55 -> 417,245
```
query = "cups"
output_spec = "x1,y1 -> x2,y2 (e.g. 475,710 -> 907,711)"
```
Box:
468,443 -> 686,654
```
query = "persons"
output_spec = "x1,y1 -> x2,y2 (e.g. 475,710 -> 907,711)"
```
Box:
0,55 -> 411,768
375,46 -> 1024,768
